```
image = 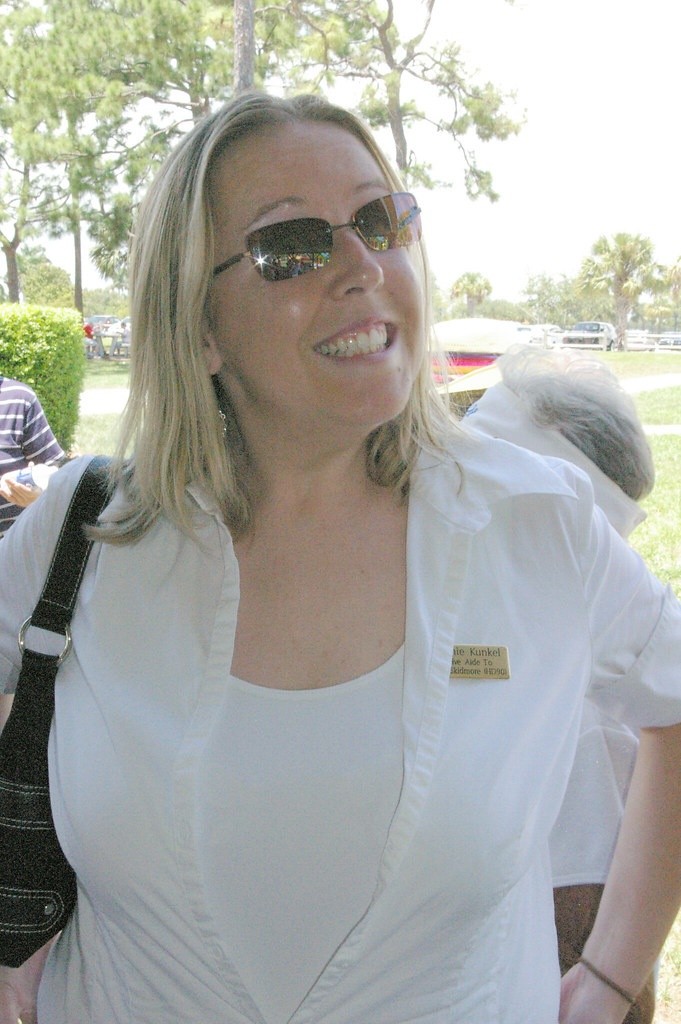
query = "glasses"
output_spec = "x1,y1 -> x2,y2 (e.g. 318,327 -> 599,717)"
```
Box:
208,193 -> 422,286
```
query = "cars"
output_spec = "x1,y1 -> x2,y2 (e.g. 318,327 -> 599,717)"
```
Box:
531,321 -> 681,353
85,313 -> 131,338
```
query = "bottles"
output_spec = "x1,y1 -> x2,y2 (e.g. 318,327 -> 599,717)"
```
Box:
0,463 -> 58,497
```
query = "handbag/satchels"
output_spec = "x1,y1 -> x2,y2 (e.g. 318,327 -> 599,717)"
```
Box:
0,456 -> 125,968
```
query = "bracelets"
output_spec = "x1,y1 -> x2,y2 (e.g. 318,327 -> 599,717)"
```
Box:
577,956 -> 636,1007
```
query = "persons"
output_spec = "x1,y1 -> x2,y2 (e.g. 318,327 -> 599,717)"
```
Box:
83,321 -> 130,360
466,348 -> 655,1024
0,377 -> 65,539
0,91 -> 681,1024
